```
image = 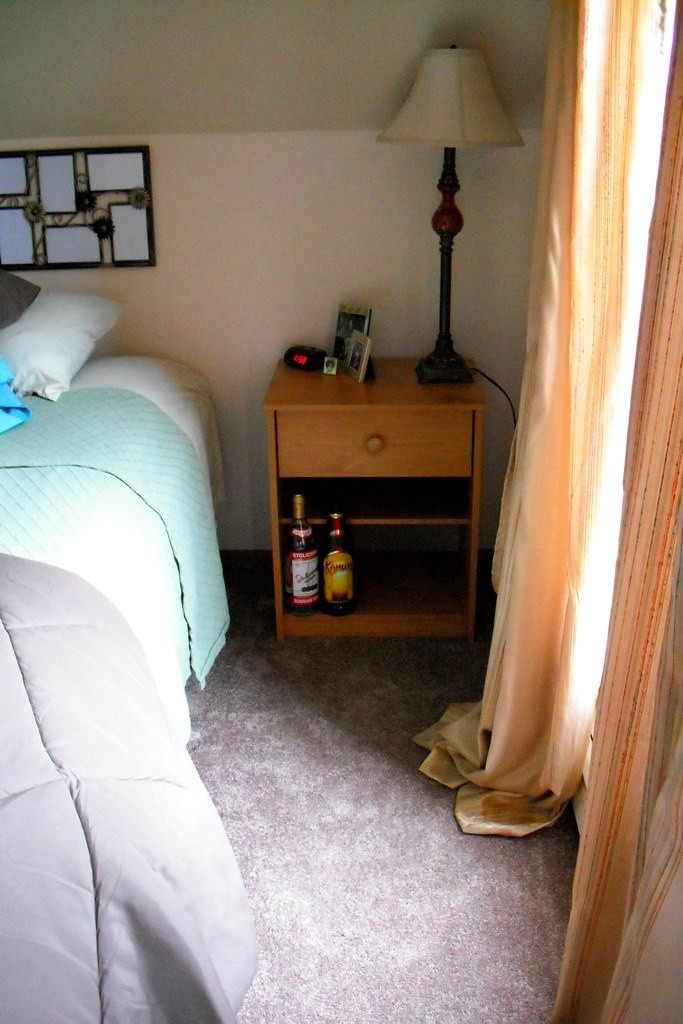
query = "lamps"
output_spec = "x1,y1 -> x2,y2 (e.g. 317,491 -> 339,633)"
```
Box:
374,42 -> 525,386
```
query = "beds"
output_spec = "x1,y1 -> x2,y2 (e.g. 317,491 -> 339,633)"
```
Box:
0,353 -> 260,1024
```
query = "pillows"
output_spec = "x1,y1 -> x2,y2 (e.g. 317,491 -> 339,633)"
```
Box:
0,269 -> 41,330
0,289 -> 124,402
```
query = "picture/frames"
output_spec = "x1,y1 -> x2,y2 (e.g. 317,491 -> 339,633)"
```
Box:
332,304 -> 372,368
344,329 -> 374,383
0,145 -> 156,271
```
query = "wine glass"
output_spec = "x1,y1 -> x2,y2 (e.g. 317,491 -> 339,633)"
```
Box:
285,494 -> 321,616
321,513 -> 356,616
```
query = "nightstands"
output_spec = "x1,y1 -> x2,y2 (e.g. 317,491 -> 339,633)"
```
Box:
261,357 -> 487,645
